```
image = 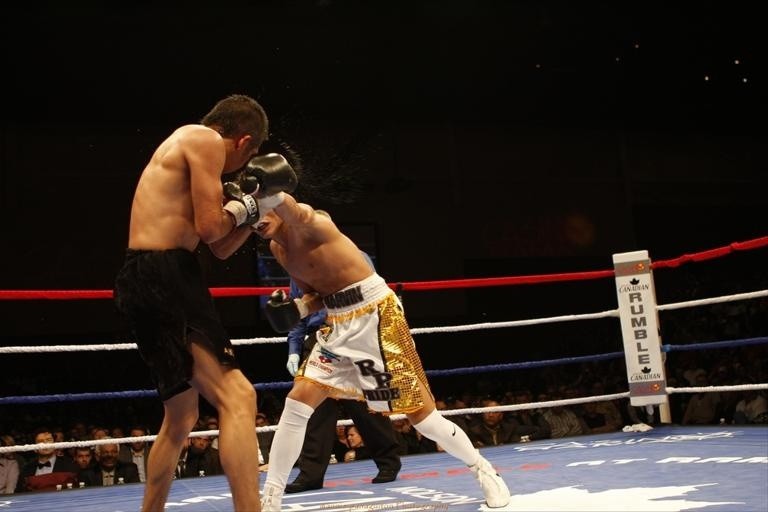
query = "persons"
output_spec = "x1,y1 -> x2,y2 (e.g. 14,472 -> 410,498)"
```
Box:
244,185 -> 514,511
111,93 -> 300,510
433,355 -> 768,448
1,400 -> 442,490
281,278 -> 402,493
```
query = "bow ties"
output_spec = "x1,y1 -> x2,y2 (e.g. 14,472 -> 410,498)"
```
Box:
38,460 -> 52,470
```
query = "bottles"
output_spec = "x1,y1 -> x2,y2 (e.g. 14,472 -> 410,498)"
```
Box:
329,454 -> 337,463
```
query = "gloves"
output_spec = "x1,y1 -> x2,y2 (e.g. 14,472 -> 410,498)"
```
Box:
264,288 -> 308,333
221,151 -> 301,209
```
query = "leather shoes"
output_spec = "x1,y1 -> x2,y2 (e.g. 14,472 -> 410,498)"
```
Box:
283,476 -> 326,496
368,463 -> 402,486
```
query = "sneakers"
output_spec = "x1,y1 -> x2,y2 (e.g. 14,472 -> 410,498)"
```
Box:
468,453 -> 513,507
